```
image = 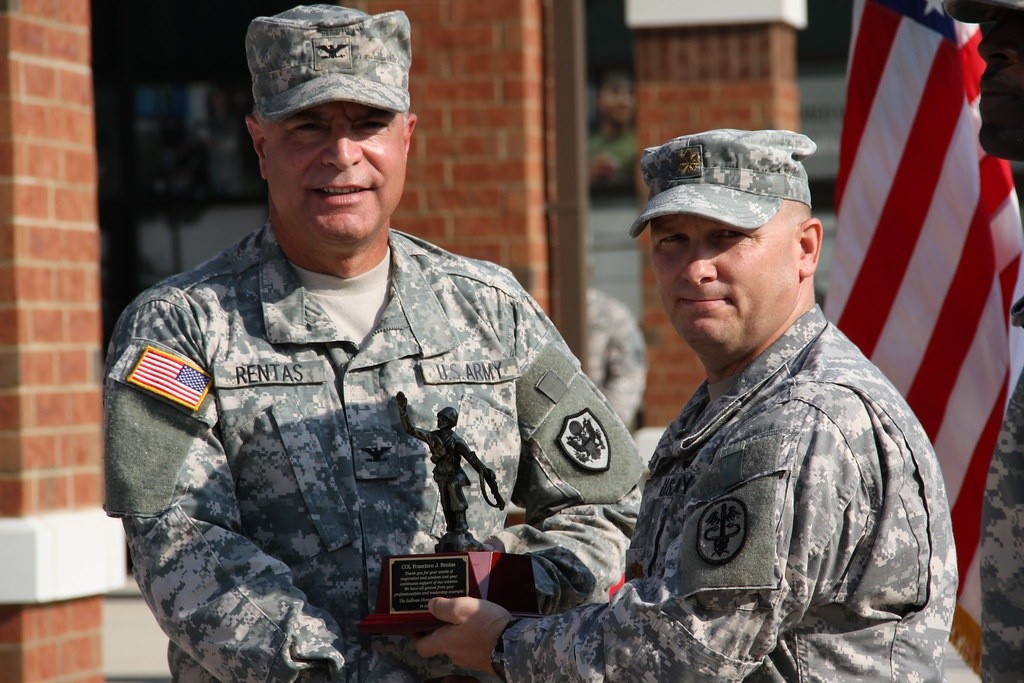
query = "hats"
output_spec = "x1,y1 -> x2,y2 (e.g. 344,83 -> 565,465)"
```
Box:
245,5 -> 412,123
630,129 -> 817,238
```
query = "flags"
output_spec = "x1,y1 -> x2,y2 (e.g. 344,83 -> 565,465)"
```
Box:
819,0 -> 1023,681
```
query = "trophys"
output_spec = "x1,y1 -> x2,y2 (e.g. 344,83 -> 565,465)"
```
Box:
356,391 -> 545,634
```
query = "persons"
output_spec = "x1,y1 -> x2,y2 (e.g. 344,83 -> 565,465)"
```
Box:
102,5 -> 651,683
584,288 -> 649,429
412,129 -> 959,683
944,0 -> 1024,683
585,68 -> 638,200
